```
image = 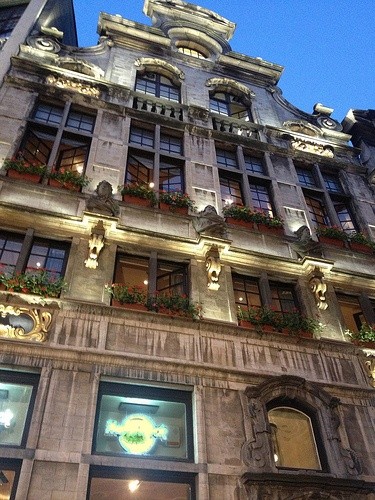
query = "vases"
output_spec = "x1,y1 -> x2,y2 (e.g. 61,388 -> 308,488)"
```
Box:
159,202 -> 188,217
226,217 -> 254,229
241,320 -> 313,338
8,169 -> 41,183
350,242 -> 373,254
124,195 -> 151,207
352,339 -> 375,349
318,236 -> 344,247
50,178 -> 80,193
112,298 -> 192,318
258,223 -> 283,236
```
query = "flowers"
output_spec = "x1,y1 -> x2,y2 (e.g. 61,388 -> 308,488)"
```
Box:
344,321 -> 375,342
2,151 -> 49,176
221,202 -> 256,221
314,225 -> 350,241
117,181 -> 157,203
236,303 -> 325,334
255,209 -> 285,227
348,230 -> 375,247
103,282 -> 202,313
0,264 -> 71,299
48,166 -> 93,186
157,189 -> 198,212
